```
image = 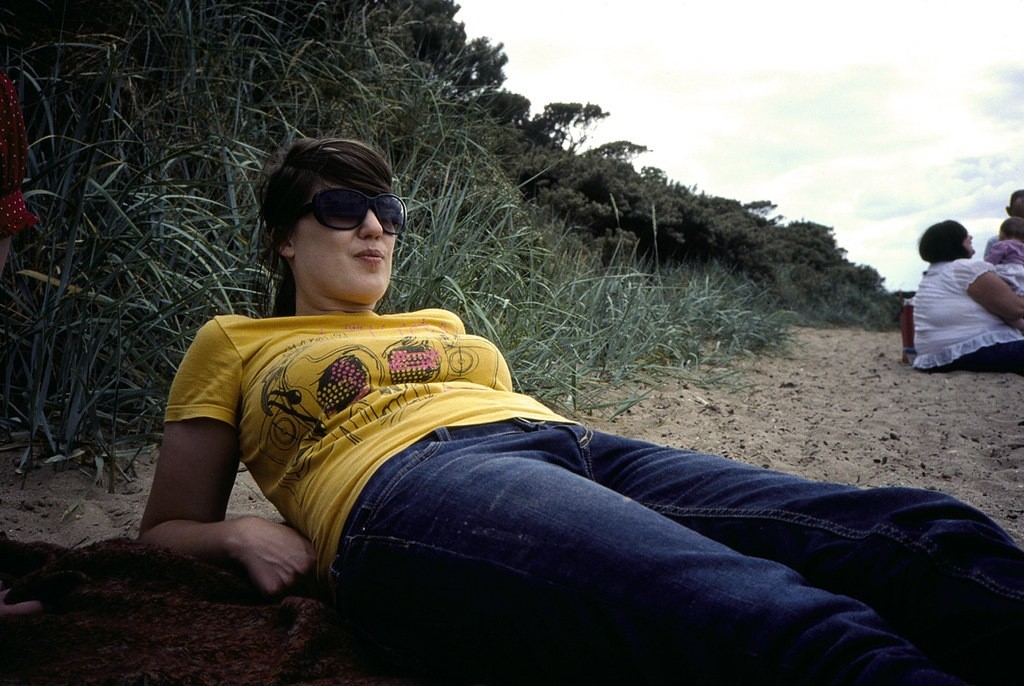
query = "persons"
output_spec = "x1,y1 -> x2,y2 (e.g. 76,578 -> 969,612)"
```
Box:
0,68 -> 38,290
984,190 -> 1024,261
912,219 -> 1024,374
984,218 -> 1024,297
138,136 -> 1024,686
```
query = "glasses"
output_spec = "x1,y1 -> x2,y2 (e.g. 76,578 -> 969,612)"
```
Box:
300,187 -> 407,236
